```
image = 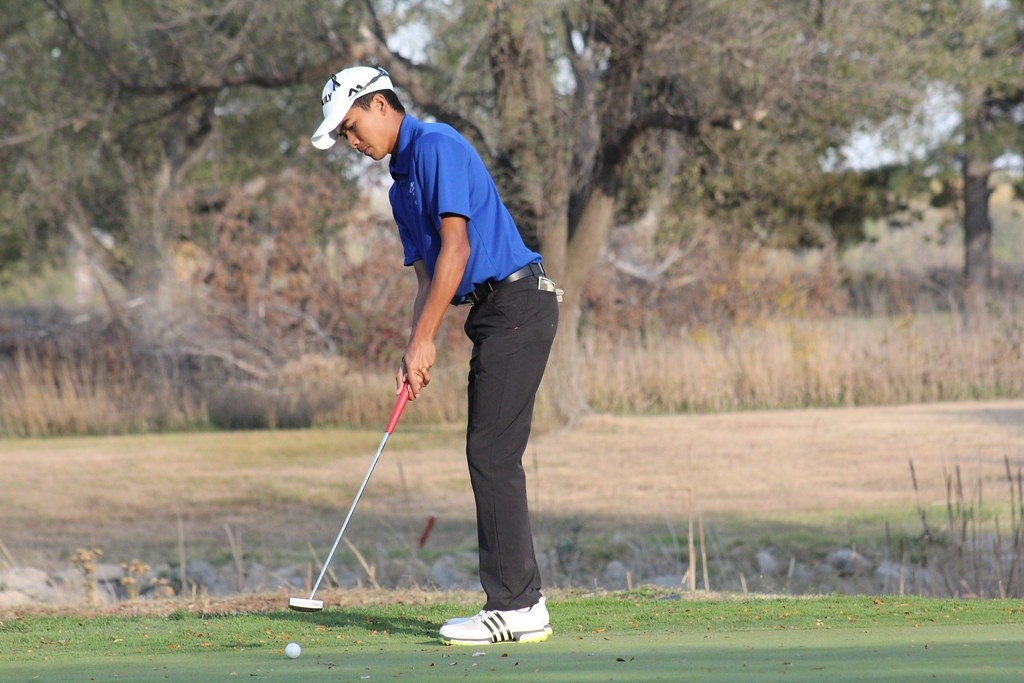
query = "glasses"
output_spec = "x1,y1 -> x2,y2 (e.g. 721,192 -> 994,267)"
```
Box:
349,65 -> 390,102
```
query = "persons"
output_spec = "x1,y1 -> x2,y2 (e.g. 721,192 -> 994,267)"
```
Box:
310,66 -> 558,647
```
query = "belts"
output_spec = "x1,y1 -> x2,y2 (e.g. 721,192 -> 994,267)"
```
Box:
464,262 -> 545,306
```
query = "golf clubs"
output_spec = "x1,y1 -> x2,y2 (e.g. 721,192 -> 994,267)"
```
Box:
288,380 -> 410,612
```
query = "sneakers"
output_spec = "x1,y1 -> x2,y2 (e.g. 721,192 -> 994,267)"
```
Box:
438,603 -> 551,646
446,595 -> 553,645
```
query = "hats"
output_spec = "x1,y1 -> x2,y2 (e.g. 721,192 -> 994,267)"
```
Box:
311,66 -> 394,150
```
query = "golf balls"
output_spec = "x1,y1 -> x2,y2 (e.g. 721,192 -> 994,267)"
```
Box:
285,643 -> 302,659
